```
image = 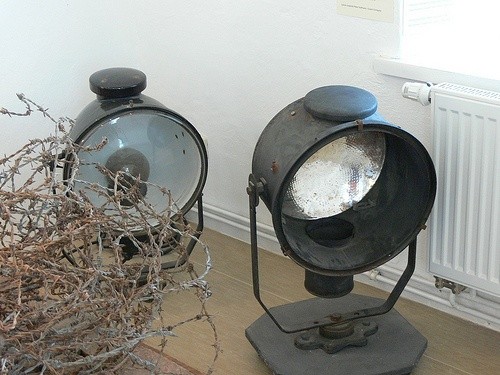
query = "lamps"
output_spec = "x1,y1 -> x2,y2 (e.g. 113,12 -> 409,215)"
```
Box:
49,68 -> 207,303
243,85 -> 437,375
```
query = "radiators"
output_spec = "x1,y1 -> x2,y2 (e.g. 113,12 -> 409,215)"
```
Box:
401,81 -> 500,298
